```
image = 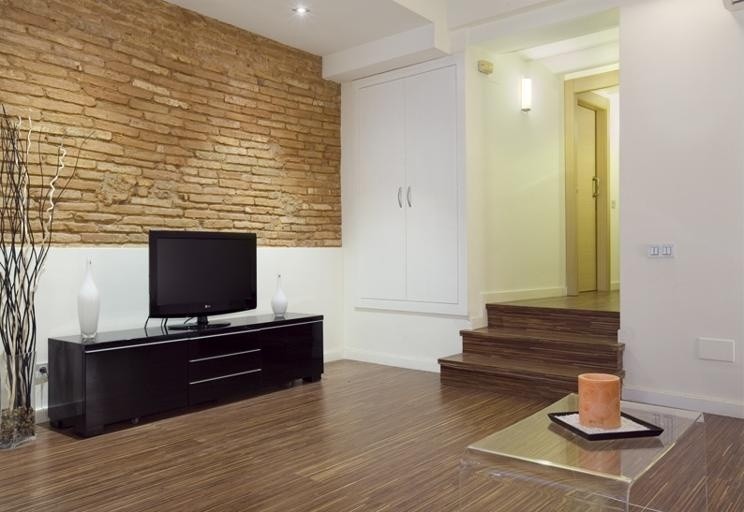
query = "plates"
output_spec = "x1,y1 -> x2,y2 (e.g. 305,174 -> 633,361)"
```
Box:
547,410 -> 665,443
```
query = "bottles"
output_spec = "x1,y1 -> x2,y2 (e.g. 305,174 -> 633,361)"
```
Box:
270,273 -> 289,319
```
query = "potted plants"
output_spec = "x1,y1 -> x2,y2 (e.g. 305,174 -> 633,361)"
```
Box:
0,101 -> 98,451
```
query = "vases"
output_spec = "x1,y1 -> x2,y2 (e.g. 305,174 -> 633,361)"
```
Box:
271,274 -> 287,318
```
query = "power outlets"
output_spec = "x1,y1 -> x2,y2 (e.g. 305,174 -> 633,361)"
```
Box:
35,361 -> 48,383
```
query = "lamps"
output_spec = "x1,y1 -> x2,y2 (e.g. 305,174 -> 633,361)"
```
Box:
521,78 -> 533,112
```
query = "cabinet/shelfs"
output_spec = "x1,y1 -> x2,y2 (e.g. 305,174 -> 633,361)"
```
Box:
48,311 -> 324,438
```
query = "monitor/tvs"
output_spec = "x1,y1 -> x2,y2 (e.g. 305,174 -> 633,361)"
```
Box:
148,229 -> 257,329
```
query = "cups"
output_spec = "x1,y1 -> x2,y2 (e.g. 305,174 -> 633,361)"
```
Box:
576,372 -> 623,430
